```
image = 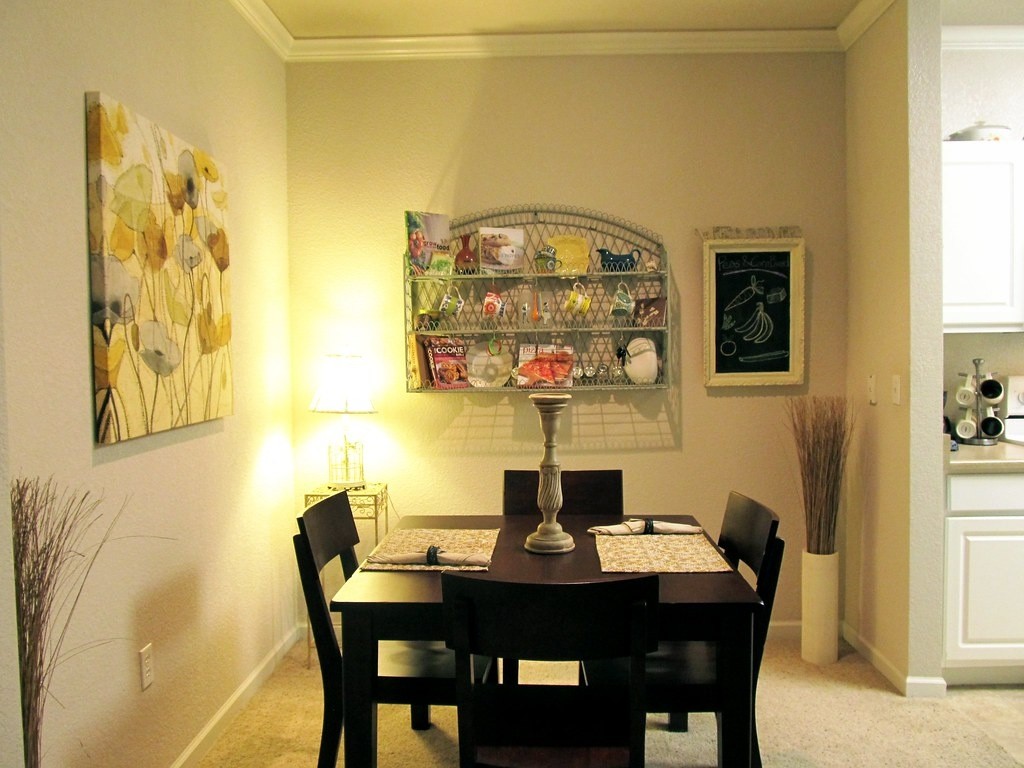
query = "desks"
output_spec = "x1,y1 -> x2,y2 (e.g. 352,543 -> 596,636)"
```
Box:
330,514 -> 765,768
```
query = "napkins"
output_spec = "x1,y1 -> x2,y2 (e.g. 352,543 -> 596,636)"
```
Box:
586,518 -> 703,536
366,546 -> 491,567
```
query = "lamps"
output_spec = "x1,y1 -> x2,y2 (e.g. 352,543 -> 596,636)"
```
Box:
306,353 -> 382,490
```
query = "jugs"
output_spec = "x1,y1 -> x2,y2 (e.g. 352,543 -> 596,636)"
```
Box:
596,248 -> 641,272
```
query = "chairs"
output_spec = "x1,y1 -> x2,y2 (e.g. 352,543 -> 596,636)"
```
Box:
292,469 -> 785,768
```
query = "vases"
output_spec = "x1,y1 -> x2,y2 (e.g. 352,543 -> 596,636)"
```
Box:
781,550 -> 851,664
524,393 -> 575,554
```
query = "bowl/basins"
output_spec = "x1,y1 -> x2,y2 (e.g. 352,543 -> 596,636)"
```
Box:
482,292 -> 507,320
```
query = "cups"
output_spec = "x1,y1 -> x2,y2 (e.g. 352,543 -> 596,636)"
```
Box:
438,285 -> 465,318
564,282 -> 591,317
979,373 -> 1004,405
609,282 -> 636,318
980,407 -> 1004,438
535,257 -> 562,274
955,374 -> 976,408
955,409 -> 977,439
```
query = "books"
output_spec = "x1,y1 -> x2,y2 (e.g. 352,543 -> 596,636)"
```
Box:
404,211 -> 575,389
633,297 -> 667,327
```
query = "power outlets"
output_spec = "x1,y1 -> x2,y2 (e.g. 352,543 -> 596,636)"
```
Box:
140,643 -> 154,690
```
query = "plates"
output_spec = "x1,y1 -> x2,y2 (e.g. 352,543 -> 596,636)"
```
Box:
465,341 -> 513,387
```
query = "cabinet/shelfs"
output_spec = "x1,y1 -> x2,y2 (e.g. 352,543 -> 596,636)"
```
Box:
942,142 -> 1024,686
305,483 -> 389,669
405,202 -> 672,391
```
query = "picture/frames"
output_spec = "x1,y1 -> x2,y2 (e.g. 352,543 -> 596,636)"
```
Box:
703,238 -> 805,386
